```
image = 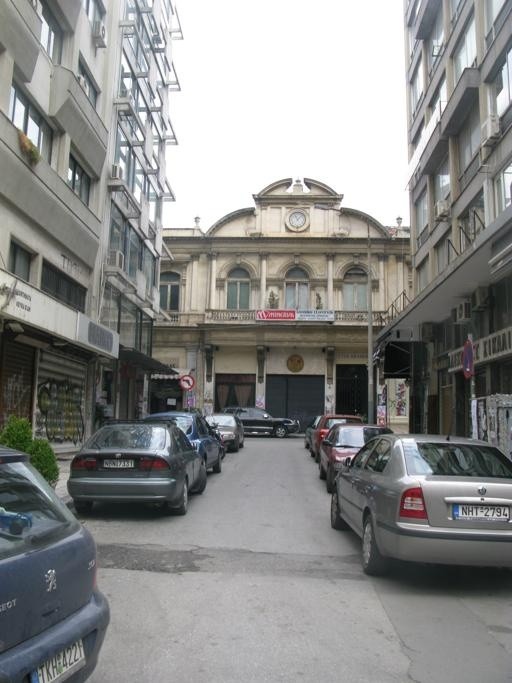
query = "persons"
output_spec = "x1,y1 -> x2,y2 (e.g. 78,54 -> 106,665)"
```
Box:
315,291 -> 322,309
268,290 -> 276,309
378,405 -> 385,425
382,385 -> 387,402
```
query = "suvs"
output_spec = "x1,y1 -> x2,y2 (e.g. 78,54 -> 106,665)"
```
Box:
219,407 -> 298,438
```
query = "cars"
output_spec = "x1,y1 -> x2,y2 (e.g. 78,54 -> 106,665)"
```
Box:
0,446 -> 109,683
331,432 -> 511,575
305,415 -> 364,462
203,413 -> 244,452
142,411 -> 222,475
319,422 -> 395,492
67,422 -> 207,515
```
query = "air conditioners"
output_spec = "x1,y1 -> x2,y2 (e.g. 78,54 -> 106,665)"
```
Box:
433,198 -> 450,223
79,74 -> 90,95
108,249 -> 126,270
479,112 -> 501,146
94,18 -> 109,48
111,163 -> 124,179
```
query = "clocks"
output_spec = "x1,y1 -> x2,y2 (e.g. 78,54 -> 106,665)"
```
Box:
284,207 -> 310,231
